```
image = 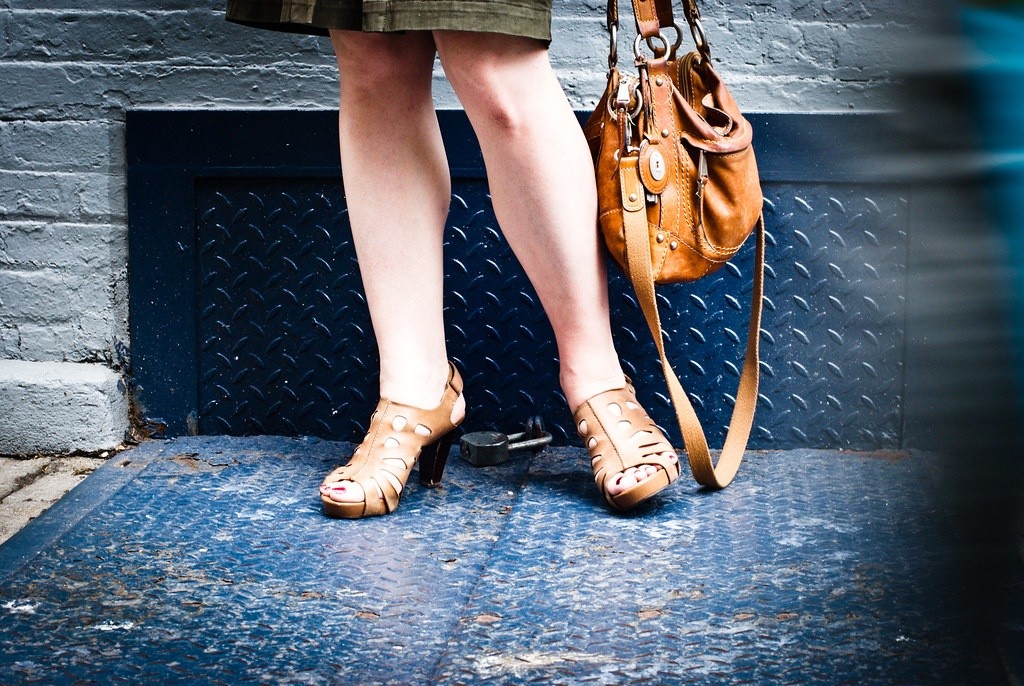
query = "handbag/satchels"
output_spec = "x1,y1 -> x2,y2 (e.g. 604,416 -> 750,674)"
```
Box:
584,0 -> 765,489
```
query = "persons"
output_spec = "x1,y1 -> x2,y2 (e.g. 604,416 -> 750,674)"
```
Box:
223,0 -> 684,518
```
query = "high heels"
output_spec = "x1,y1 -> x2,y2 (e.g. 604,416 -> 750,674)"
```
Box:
573,374 -> 681,511
322,360 -> 463,517
